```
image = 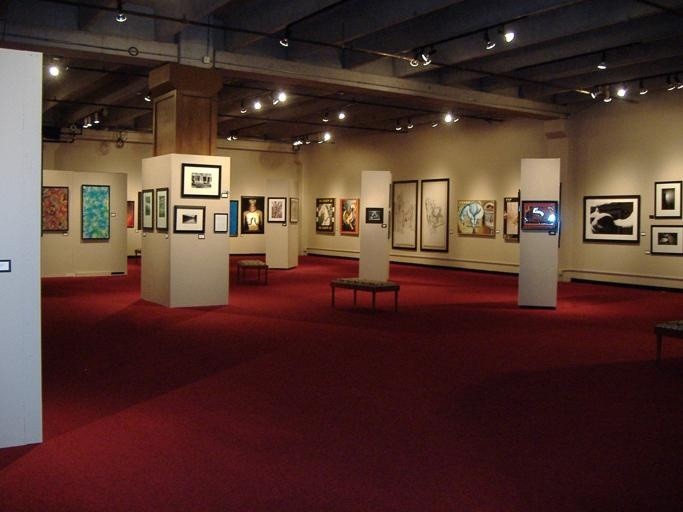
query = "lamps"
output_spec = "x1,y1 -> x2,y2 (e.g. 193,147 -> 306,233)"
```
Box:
409,45 -> 438,66
497,22 -> 514,43
114,3 -> 127,23
395,111 -> 458,132
82,111 -> 100,128
279,38 -> 289,47
589,70 -> 683,103
225,85 -> 346,148
597,50 -> 607,69
144,89 -> 153,102
482,26 -> 496,51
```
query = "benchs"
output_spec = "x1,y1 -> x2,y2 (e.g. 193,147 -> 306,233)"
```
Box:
235,258 -> 269,285
329,277 -> 401,314
654,320 -> 683,360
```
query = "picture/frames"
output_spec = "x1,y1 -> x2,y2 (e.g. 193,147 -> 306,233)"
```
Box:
127,201 -> 134,227
582,194 -> 640,243
339,198 -> 360,234
521,201 -> 557,232
42,186 -> 69,232
155,188 -> 169,231
654,181 -> 682,220
174,204 -> 206,232
267,197 -> 286,223
229,200 -> 238,236
213,213 -> 227,232
181,163 -> 222,199
142,189 -> 153,229
240,195 -> 264,234
391,179 -> 418,249
290,197 -> 298,222
315,198 -> 335,233
650,224 -> 683,256
138,192 -> 141,229
365,208 -> 384,223
81,184 -> 110,240
420,178 -> 450,252
503,197 -> 518,240
